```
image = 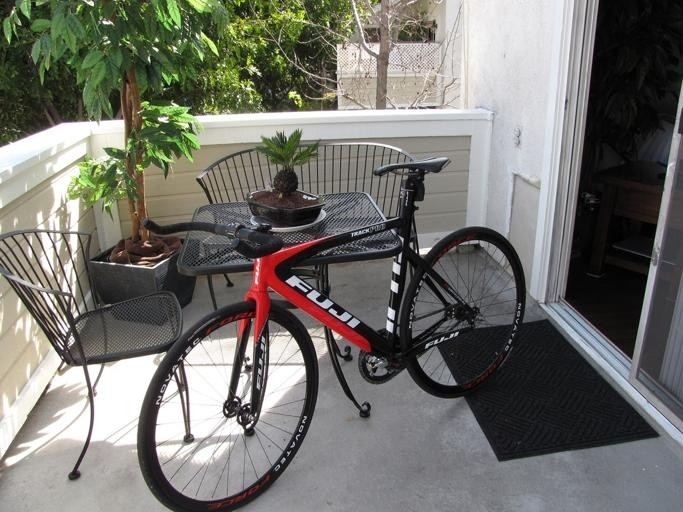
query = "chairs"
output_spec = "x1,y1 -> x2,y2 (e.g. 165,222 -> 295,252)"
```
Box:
0,226 -> 193,478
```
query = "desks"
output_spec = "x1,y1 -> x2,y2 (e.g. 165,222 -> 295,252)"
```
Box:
584,160 -> 683,299
176,192 -> 404,423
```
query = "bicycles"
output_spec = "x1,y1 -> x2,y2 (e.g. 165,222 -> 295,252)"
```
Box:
135,156 -> 529,512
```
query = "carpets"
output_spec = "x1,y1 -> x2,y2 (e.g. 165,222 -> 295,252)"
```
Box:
438,317 -> 660,459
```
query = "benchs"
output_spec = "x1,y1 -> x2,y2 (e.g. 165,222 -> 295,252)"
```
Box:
195,140 -> 416,313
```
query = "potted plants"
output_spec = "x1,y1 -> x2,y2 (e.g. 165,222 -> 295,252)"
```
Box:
246,128 -> 325,229
0,0 -> 228,320
